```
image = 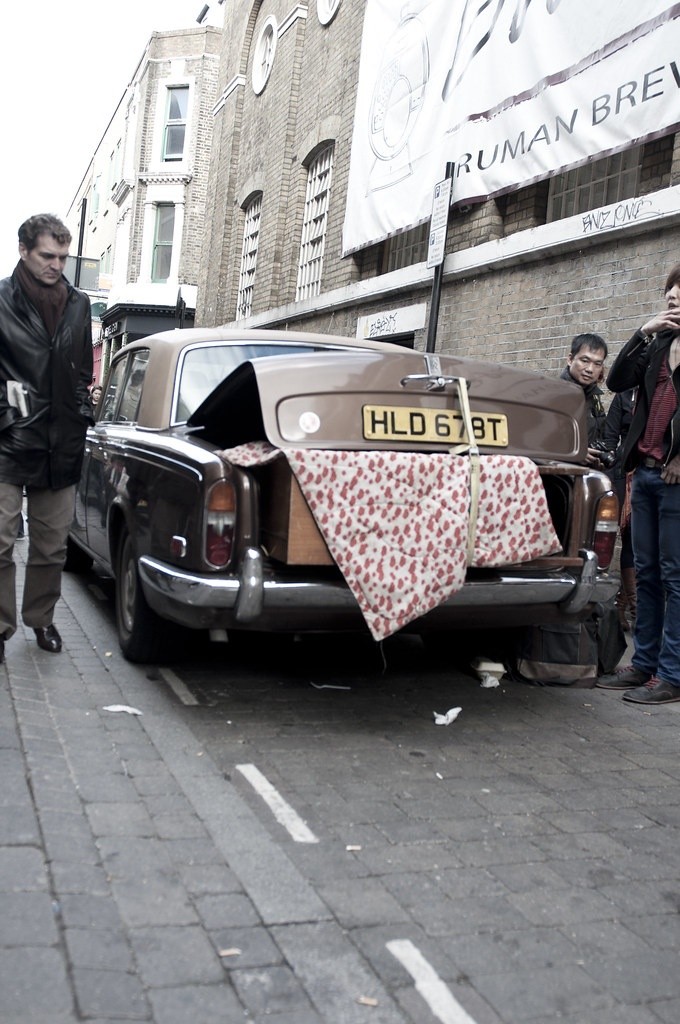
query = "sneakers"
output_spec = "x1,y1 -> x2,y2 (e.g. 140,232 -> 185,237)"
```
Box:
596,665 -> 650,689
623,676 -> 680,703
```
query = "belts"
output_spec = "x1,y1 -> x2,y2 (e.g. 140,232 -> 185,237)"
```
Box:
639,456 -> 663,470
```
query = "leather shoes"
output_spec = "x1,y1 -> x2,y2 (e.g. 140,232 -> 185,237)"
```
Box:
34,624 -> 62,651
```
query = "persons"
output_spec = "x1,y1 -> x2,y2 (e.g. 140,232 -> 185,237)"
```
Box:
555,334 -> 642,633
0,214 -> 97,658
596,265 -> 680,705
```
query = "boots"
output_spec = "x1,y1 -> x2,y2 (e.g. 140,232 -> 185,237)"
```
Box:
621,567 -> 637,630
614,586 -> 629,631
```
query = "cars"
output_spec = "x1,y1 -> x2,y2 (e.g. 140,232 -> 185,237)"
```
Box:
57,327 -> 619,667
